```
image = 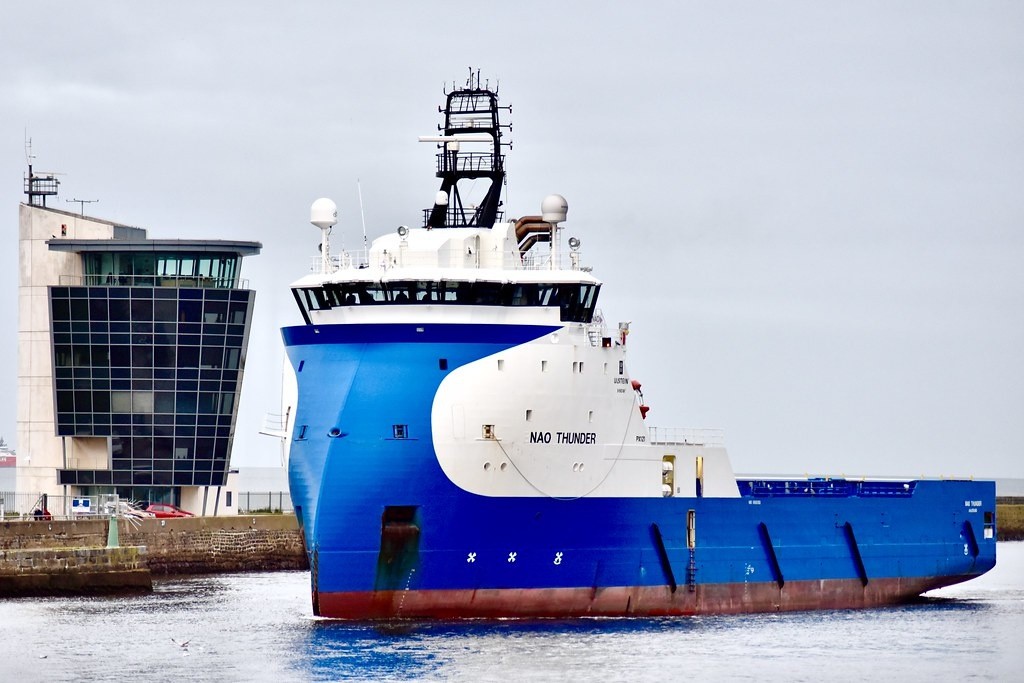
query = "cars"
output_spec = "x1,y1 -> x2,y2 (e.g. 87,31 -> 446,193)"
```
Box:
145,503 -> 194,518
106,501 -> 156,519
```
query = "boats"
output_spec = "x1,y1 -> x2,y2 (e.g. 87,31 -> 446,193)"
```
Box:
279,68 -> 996,623
